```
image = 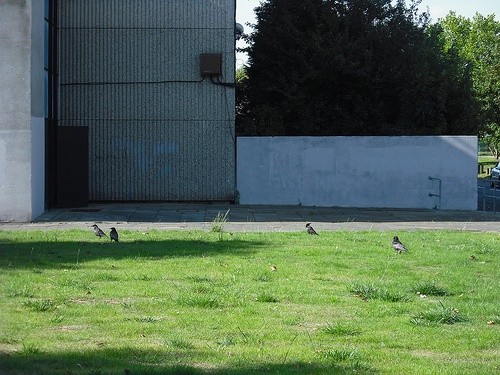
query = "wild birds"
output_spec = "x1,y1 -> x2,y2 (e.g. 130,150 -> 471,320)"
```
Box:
392,235 -> 409,254
305,222 -> 319,236
91,224 -> 106,239
108,227 -> 119,243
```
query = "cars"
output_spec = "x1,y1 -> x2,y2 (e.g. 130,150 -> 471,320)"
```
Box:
490,160 -> 500,188
